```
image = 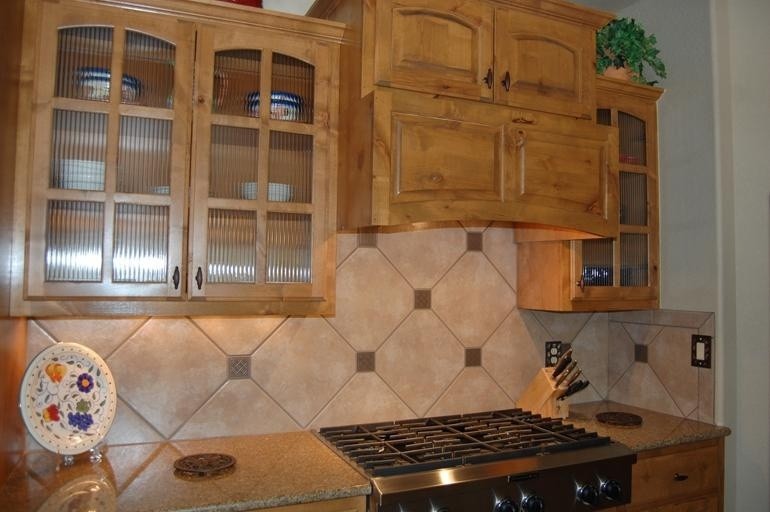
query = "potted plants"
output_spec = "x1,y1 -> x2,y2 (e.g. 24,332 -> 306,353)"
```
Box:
594,17 -> 667,87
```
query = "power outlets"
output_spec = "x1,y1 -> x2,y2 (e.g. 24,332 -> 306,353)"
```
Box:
546,340 -> 561,365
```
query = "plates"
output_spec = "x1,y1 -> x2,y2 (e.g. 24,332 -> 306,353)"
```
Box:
57,160 -> 105,191
242,182 -> 294,202
20,342 -> 118,456
152,186 -> 170,195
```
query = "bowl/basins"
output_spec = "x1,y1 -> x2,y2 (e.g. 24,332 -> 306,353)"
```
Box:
248,91 -> 303,120
78,66 -> 141,104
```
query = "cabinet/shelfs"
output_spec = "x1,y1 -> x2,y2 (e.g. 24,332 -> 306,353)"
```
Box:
0,0 -> 346,318
516,75 -> 665,313
618,434 -> 724,512
338,87 -> 620,243
304,0 -> 620,121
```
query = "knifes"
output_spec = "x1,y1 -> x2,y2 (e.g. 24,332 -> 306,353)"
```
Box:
555,349 -> 589,400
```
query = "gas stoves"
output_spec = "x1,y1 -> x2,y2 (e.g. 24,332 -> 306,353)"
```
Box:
321,406 -> 638,512
